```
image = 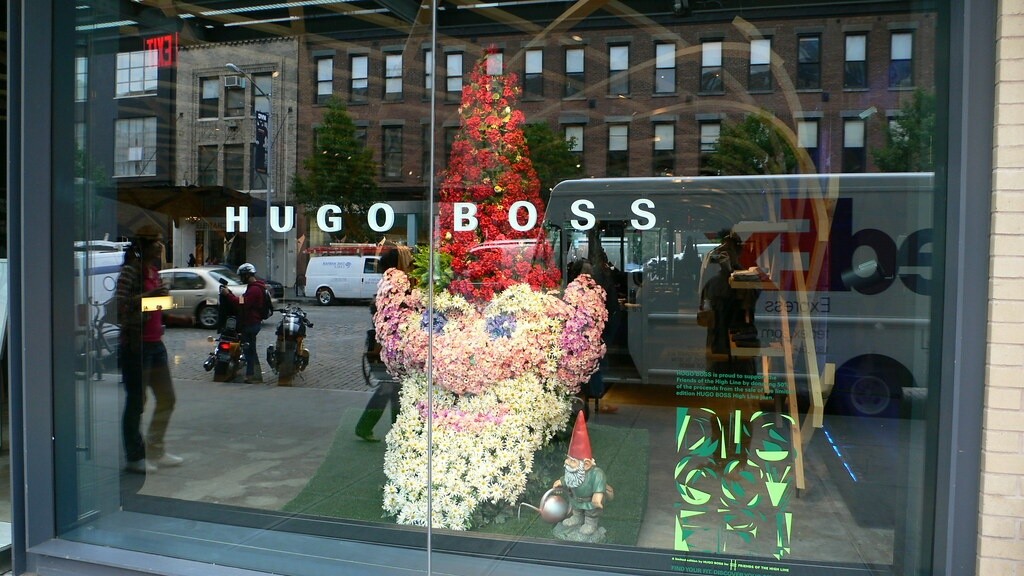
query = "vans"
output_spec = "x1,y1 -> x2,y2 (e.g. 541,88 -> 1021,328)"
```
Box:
454,236 -> 628,295
304,255 -> 383,306
683,243 -> 720,259
75,239 -> 128,362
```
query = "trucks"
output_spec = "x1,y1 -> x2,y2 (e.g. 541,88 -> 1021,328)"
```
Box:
528,173 -> 933,418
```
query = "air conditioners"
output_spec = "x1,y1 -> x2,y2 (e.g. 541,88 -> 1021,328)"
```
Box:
224,76 -> 241,87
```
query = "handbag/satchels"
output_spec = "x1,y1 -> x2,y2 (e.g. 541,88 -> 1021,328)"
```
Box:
359,330 -> 392,380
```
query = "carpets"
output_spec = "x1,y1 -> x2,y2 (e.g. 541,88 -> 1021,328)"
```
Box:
281,407 -> 653,547
802,414 -> 903,528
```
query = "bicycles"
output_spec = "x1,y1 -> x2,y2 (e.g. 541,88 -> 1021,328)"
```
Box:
75,296 -> 123,380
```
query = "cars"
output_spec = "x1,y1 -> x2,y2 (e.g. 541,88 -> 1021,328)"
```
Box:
158,267 -> 279,327
630,256 -> 666,274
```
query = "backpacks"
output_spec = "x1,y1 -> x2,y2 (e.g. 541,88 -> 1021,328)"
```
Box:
254,285 -> 273,320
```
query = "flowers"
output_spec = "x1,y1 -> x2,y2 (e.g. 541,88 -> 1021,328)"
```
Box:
371,42 -> 610,530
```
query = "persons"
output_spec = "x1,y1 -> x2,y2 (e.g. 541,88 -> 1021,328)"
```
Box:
220,263 -> 269,383
187,254 -> 217,266
354,245 -> 416,442
570,232 -> 760,479
116,225 -> 184,473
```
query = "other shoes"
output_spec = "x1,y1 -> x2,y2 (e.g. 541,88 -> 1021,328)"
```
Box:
244,374 -> 263,382
147,453 -> 184,467
124,460 -> 158,474
354,429 -> 381,442
724,461 -> 746,481
696,458 -> 729,478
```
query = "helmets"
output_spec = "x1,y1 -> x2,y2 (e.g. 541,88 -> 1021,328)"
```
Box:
236,263 -> 255,274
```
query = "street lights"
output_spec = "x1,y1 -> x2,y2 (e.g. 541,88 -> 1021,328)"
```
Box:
226,63 -> 272,278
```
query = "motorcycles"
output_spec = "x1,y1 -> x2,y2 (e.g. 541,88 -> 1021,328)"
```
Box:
266,304 -> 314,386
202,277 -> 250,381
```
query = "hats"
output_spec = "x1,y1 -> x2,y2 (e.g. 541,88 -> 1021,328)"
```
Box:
705,229 -> 742,252
